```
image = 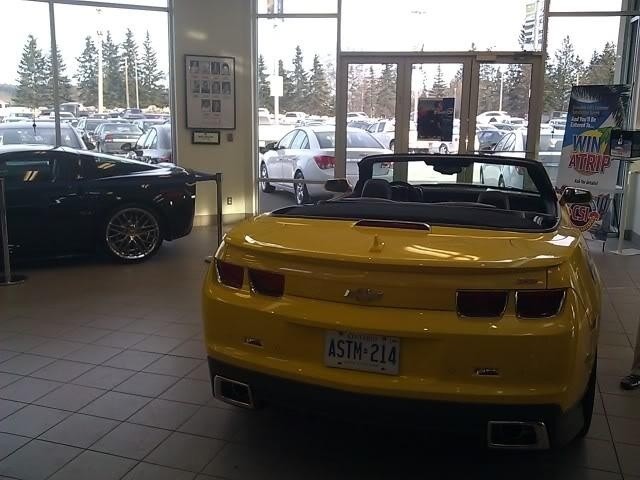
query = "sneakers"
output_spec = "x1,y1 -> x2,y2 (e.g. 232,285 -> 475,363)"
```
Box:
620,374 -> 640,390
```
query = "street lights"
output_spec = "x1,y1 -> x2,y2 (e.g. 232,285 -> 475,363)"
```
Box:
96,9 -> 103,114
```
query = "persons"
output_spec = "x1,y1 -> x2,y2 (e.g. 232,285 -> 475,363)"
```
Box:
620,325 -> 640,391
430,102 -> 446,129
191,62 -> 230,112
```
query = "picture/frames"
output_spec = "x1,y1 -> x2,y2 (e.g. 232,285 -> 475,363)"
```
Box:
193,131 -> 221,145
184,54 -> 236,129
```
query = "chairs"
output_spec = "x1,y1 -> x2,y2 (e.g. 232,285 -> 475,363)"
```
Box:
360,179 -> 392,200
476,191 -> 511,211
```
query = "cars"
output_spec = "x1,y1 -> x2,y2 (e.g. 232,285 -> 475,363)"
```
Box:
0,102 -> 196,264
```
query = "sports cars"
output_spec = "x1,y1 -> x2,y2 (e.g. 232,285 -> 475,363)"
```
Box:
202,153 -> 602,458
